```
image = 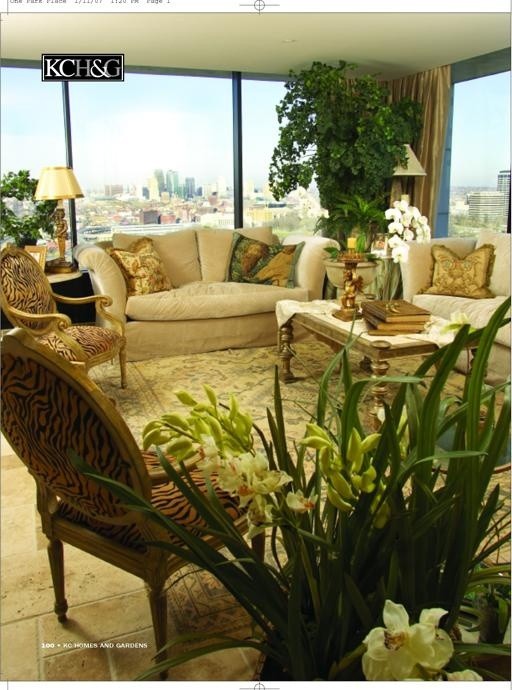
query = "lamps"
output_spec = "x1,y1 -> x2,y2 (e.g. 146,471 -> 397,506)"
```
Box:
388,142 -> 426,205
34,167 -> 85,273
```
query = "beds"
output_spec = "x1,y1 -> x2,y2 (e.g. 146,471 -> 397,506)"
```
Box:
40,273 -> 83,322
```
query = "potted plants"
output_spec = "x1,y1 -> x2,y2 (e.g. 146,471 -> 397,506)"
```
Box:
312,192 -> 392,305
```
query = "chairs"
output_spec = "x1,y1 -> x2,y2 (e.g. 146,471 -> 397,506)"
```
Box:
0,326 -> 266,679
0,243 -> 128,389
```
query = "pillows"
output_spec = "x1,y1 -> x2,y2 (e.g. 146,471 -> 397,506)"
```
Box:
111,227 -> 201,289
196,225 -> 273,282
417,244 -> 495,297
222,231 -> 305,290
104,236 -> 175,295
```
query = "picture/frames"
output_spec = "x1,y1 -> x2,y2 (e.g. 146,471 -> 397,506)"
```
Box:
23,246 -> 48,274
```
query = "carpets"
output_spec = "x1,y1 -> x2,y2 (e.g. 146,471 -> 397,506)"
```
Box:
70,296 -> 511,682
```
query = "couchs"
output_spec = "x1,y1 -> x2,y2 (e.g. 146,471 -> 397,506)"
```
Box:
72,223 -> 341,366
401,236 -> 512,349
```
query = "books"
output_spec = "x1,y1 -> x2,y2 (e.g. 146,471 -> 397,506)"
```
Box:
361,298 -> 431,336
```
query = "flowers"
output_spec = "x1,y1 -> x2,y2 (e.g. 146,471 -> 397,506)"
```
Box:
385,201 -> 431,300
